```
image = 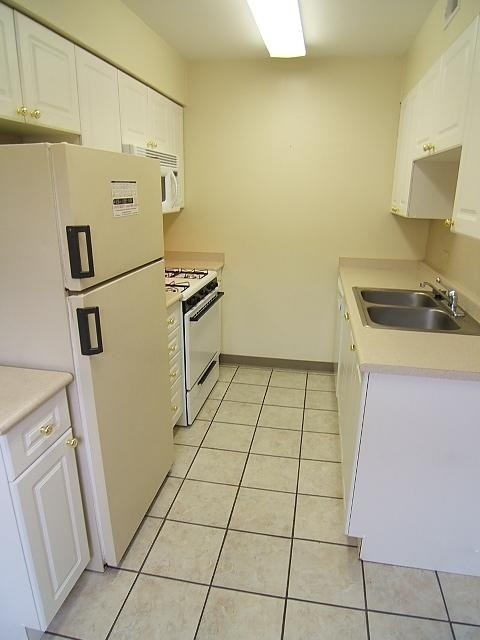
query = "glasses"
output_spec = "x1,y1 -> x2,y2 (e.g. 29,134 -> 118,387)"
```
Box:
249,0 -> 308,62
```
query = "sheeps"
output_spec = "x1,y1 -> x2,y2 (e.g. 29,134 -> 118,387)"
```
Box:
420,280 -> 464,317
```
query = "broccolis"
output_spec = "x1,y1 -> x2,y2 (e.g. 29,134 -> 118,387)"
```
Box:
0,141 -> 180,587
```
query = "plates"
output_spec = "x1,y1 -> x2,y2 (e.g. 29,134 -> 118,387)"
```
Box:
165,298 -> 185,432
165,97 -> 187,210
75,44 -> 123,152
388,20 -> 479,241
1,388 -> 93,640
117,65 -> 167,154
0,2 -> 82,138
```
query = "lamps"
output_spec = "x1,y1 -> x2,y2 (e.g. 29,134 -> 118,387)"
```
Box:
249,0 -> 308,62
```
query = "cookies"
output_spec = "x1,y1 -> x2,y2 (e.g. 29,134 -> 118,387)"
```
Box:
122,143 -> 182,214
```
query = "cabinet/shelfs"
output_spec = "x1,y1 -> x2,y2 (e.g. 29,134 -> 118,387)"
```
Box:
388,20 -> 479,241
165,97 -> 187,210
1,388 -> 93,640
117,65 -> 167,159
0,2 -> 82,138
331,268 -> 480,575
165,298 -> 185,432
75,44 -> 123,152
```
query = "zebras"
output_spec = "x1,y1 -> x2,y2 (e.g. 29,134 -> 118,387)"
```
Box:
178,287 -> 224,428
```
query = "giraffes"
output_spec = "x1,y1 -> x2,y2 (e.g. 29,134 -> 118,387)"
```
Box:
165,267 -> 219,310
